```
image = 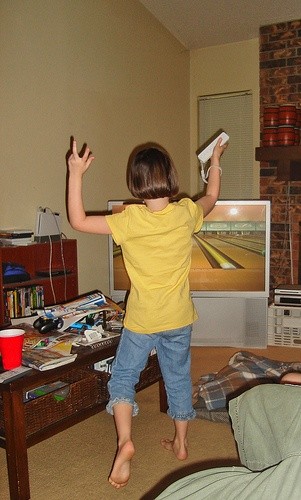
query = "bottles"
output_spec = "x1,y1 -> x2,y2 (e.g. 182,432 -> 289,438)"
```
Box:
262,103 -> 301,146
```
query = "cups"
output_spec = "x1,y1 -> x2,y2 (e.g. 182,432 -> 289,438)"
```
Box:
0,329 -> 26,371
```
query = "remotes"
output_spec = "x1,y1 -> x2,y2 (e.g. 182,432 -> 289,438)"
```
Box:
198,132 -> 229,163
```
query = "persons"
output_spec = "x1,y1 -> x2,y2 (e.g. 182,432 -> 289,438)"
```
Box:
280,372 -> 301,386
68,138 -> 229,489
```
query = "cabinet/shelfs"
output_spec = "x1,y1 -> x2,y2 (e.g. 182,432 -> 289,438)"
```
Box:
0,239 -> 78,327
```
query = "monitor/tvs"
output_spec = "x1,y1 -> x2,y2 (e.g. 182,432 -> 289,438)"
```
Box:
108,201 -> 270,298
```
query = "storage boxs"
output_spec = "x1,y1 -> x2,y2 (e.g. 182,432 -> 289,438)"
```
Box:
267,304 -> 301,348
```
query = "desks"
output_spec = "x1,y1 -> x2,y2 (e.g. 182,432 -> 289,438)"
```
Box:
0,337 -> 171,500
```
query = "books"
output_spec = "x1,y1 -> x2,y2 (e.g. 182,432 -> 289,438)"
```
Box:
0,230 -> 34,246
4,286 -> 44,319
